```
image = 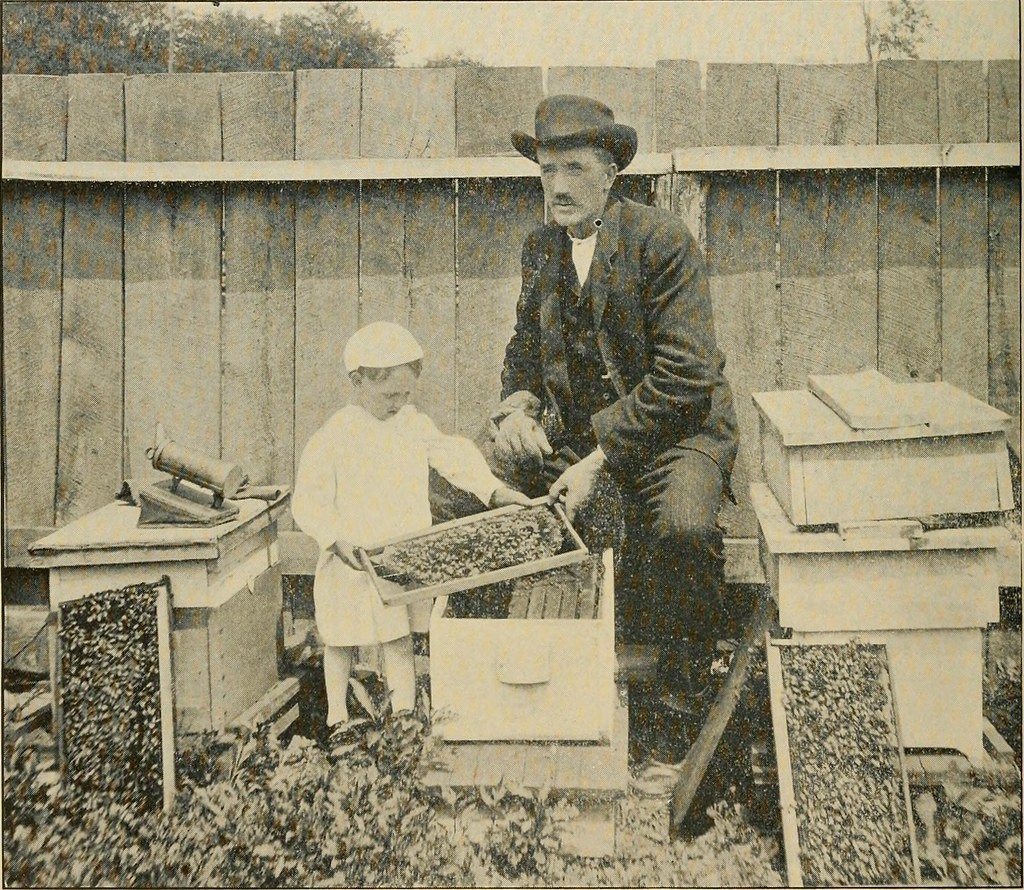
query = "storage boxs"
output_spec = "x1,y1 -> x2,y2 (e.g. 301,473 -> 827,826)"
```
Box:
747,377 -> 1017,528
787,627 -> 991,760
748,479 -> 1012,634
25,482 -> 294,745
426,542 -> 618,746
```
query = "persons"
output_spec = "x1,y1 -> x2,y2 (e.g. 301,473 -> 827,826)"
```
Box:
289,321 -> 535,762
484,94 -> 741,771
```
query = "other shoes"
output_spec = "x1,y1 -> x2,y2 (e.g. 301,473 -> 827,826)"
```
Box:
389,706 -> 419,729
325,721 -> 359,757
644,703 -> 703,765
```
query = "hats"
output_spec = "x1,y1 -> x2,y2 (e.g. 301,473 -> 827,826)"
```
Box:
343,320 -> 425,376
509,93 -> 640,171
